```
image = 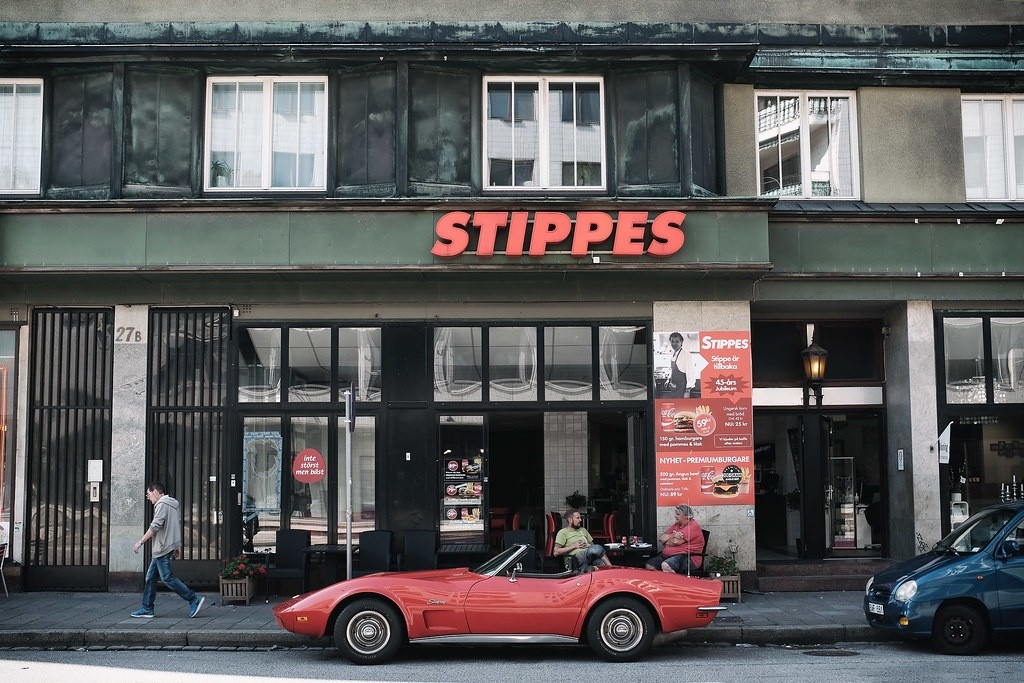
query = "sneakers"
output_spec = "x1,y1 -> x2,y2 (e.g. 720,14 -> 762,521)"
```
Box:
130,606 -> 155,618
188,596 -> 205,617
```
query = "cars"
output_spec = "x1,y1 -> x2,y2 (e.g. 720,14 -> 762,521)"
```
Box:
862,502 -> 1024,656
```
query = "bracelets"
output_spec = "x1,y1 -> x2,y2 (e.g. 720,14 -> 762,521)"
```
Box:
138,540 -> 143,546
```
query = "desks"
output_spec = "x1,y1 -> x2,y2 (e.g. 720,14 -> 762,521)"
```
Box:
301,545 -> 358,593
605,544 -> 655,566
437,544 -> 491,569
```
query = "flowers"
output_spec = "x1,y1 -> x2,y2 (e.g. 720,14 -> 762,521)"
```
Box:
707,551 -> 740,576
217,555 -> 267,580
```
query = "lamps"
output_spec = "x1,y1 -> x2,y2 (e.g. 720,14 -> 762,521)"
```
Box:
802,342 -> 829,409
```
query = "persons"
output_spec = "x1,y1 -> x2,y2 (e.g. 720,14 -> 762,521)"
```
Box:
552,508 -> 612,569
663,332 -> 696,398
130,481 -> 205,619
644,504 -> 705,576
242,496 -> 259,552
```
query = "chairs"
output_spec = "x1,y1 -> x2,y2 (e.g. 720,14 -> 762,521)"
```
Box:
397,530 -> 441,573
501,531 -> 544,574
658,529 -> 710,578
265,530 -> 311,597
351,530 -> 392,579
552,532 -> 580,572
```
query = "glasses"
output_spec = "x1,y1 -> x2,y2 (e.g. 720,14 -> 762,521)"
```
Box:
146,493 -> 152,497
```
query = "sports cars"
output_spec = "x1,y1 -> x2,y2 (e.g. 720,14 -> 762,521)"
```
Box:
270,540 -> 730,665
